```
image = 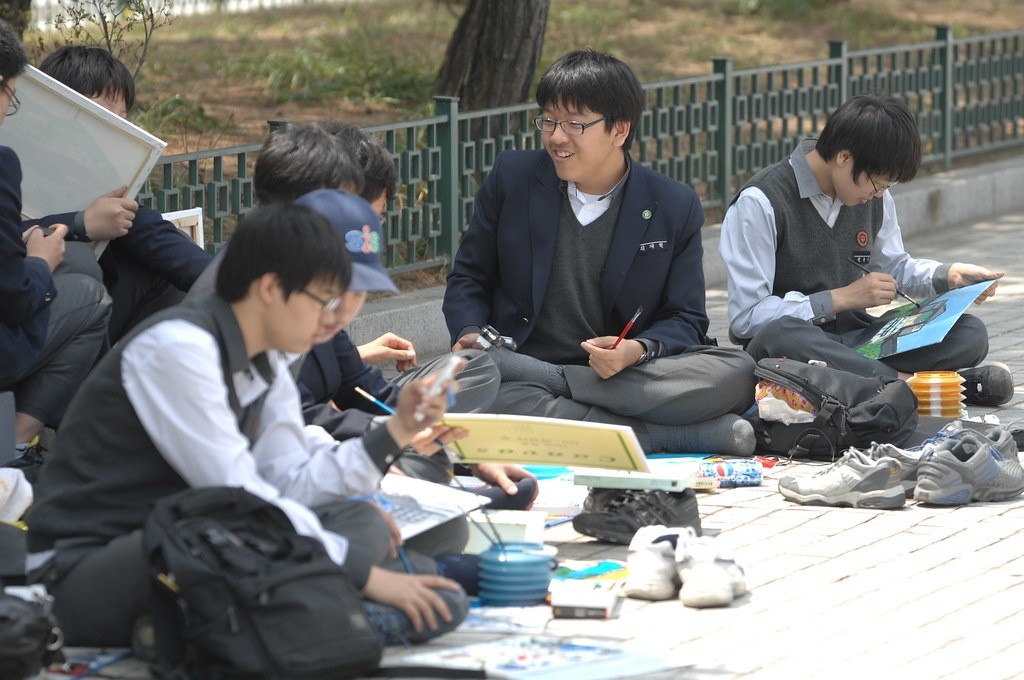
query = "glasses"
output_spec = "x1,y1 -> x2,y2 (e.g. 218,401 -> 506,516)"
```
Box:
864,168 -> 890,195
377,214 -> 386,224
534,113 -> 613,135
300,287 -> 342,311
2,79 -> 21,116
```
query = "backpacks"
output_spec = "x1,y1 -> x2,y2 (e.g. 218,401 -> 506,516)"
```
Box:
140,486 -> 384,680
0,597 -> 61,680
743,357 -> 919,462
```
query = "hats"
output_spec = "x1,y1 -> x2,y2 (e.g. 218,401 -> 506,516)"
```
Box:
293,189 -> 400,295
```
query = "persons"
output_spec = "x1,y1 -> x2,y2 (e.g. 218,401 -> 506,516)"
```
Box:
440,50 -> 758,460
23,199 -> 470,658
718,93 -> 1015,405
178,118 -> 543,577
0,0 -> 218,486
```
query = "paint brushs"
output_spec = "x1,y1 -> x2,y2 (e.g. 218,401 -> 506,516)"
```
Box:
355,385 -> 465,461
846,257 -> 922,310
610,306 -> 644,351
395,540 -> 414,574
543,517 -> 574,528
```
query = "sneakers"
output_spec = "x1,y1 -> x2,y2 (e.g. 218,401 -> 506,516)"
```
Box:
778,421 -> 1024,509
676,555 -> 746,608
4,435 -> 49,483
572,488 -> 702,544
956,362 -> 1015,407
625,542 -> 682,600
365,589 -> 467,645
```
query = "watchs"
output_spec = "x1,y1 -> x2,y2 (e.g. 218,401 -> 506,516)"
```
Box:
636,351 -> 646,364
